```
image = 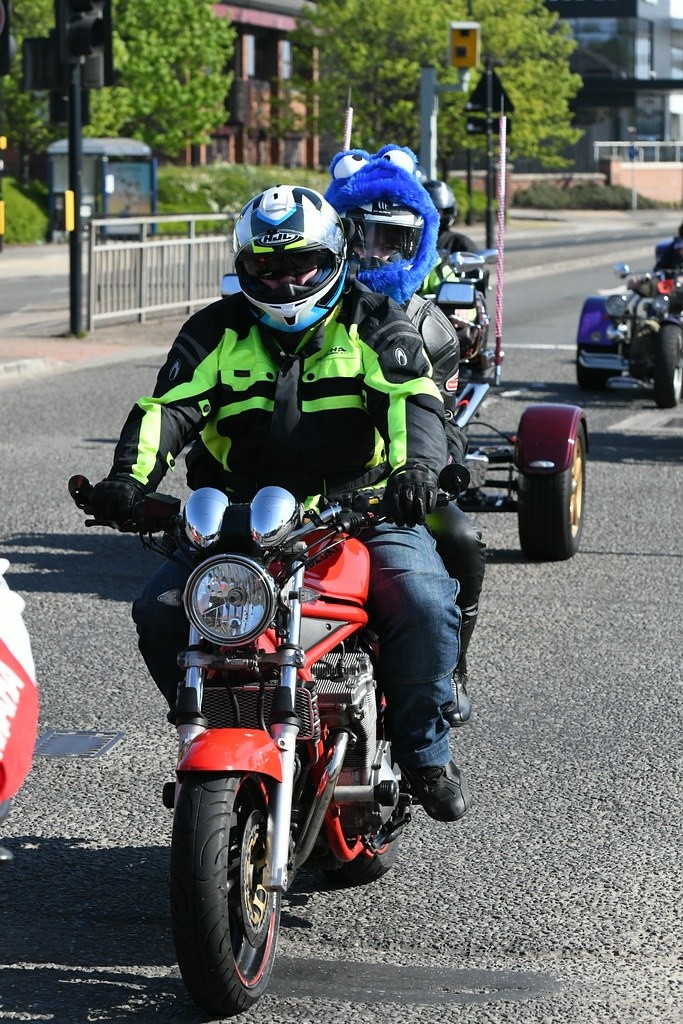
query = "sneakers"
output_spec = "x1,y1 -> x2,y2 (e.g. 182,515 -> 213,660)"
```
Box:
448,670 -> 471,726
398,759 -> 470,822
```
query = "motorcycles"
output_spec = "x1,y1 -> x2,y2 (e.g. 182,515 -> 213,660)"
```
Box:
66,458 -> 469,1017
424,244 -> 590,562
575,244 -> 683,409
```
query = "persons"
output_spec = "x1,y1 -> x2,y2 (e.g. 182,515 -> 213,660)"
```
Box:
319,143 -> 487,726
83,185 -> 471,823
420,179 -> 491,403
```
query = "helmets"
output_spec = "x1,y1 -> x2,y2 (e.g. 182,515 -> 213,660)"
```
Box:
420,178 -> 457,229
231,184 -> 348,331
338,197 -> 426,266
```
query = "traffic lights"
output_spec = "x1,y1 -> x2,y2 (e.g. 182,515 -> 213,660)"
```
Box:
53,0 -> 110,66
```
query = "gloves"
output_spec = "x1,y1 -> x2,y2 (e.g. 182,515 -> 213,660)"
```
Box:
84,479 -> 142,524
380,465 -> 438,527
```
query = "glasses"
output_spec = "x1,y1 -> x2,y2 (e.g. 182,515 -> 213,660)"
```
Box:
241,251 -> 329,280
